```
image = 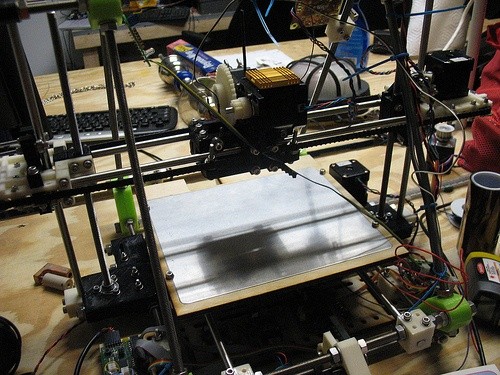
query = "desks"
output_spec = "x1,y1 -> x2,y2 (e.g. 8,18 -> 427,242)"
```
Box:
0,15 -> 500,375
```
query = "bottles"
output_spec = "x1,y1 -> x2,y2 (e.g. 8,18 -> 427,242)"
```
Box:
329,0 -> 369,67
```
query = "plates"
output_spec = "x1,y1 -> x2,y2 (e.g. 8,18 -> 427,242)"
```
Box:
0,315 -> 22,375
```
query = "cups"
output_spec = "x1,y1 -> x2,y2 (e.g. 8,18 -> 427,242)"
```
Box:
458,170 -> 500,262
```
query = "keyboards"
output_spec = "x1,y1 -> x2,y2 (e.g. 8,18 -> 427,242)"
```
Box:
139,6 -> 190,23
47,106 -> 178,147
